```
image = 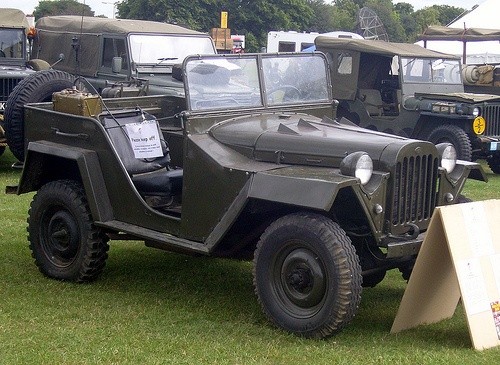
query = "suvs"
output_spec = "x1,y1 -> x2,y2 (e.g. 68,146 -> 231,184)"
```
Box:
0,6 -> 66,157
282,35 -> 500,174
420,23 -> 500,105
3,50 -> 474,342
28,14 -> 256,111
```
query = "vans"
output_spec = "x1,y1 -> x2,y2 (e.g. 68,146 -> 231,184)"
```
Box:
266,29 -> 363,52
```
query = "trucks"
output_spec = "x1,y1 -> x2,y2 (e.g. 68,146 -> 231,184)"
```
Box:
210,27 -> 246,54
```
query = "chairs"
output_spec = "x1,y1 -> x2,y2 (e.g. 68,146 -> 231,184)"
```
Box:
103,113 -> 183,209
359,89 -> 399,117
